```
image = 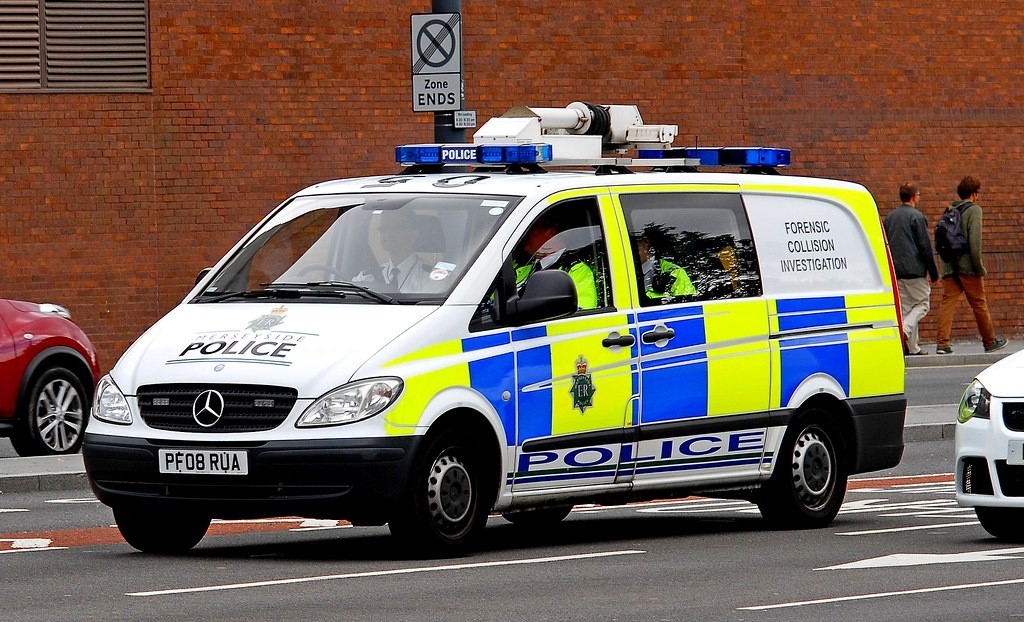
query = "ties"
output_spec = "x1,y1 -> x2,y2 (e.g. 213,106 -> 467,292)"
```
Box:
389,268 -> 400,288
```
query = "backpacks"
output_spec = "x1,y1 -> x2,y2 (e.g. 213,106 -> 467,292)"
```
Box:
933,202 -> 975,263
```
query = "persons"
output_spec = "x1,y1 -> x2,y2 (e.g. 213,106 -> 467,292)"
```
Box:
347,206 -> 456,294
883,180 -> 941,355
638,236 -> 696,305
933,175 -> 1009,354
485,213 -> 598,312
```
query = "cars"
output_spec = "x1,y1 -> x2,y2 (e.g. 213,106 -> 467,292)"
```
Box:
1,298 -> 101,457
954,348 -> 1024,543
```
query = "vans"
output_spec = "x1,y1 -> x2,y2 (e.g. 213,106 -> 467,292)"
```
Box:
81,100 -> 907,556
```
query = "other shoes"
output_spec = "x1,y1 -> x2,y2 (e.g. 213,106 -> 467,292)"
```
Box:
985,338 -> 1009,353
916,348 -> 928,354
936,346 -> 954,354
